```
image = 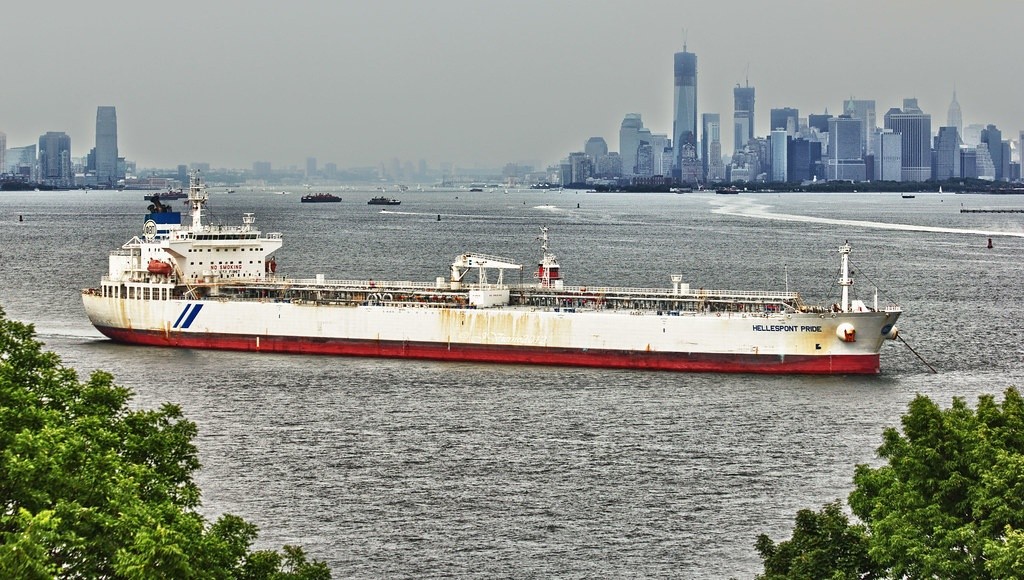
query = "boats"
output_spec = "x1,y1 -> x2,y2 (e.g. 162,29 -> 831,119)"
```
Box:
299,190 -> 342,202
77,173 -> 905,375
367,195 -> 401,206
142,189 -> 188,200
